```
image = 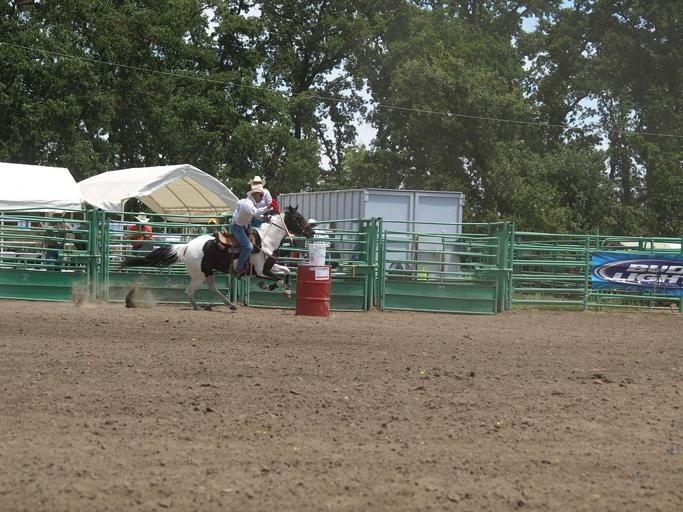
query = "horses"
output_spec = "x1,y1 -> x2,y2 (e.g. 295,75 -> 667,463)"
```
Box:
117,203 -> 315,311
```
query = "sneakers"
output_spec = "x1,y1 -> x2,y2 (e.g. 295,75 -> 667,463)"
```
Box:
235,272 -> 247,282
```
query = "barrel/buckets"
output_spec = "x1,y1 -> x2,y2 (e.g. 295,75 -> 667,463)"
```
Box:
295,264 -> 333,316
308,244 -> 326,266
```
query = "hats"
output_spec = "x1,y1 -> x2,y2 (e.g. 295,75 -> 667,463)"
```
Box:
247,175 -> 267,197
208,218 -> 217,224
48,211 -> 66,218
134,212 -> 151,222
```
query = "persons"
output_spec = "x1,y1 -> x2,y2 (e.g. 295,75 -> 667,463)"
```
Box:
244,175 -> 273,226
123,211 -> 151,257
229,184 -> 273,283
36,209 -> 72,272
